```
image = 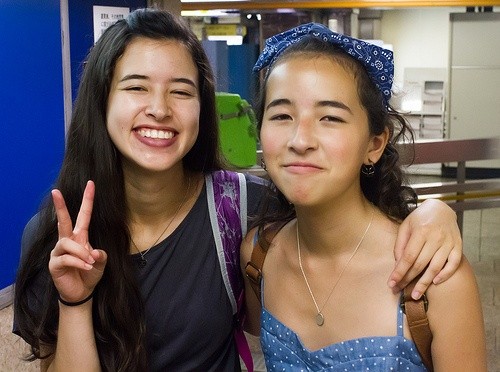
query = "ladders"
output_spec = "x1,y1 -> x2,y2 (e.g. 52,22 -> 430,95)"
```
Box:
419,80 -> 446,140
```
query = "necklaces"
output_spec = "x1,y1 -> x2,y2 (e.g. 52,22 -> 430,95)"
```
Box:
296,203 -> 376,326
126,176 -> 191,268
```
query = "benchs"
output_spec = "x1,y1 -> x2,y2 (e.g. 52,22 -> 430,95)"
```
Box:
386,112 -> 445,175
251,138 -> 500,243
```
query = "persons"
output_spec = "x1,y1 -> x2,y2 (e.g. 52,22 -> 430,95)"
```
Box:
238,20 -> 486,371
11,7 -> 462,372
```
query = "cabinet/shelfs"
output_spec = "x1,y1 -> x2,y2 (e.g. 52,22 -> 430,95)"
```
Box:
442,13 -> 500,178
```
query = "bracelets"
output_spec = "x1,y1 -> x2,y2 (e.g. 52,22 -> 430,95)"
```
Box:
57,295 -> 94,307
